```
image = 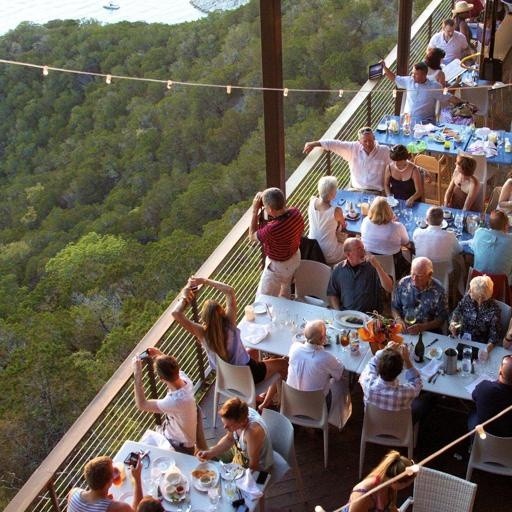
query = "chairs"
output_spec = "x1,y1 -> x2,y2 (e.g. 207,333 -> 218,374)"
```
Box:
395,464 -> 478,512
358,401 -> 419,484
278,382 -> 333,471
212,356 -> 279,429
259,407 -> 307,512
291,240 -> 510,341
464,429 -> 512,484
194,408 -> 208,450
401,85 -> 490,128
414,155 -> 511,214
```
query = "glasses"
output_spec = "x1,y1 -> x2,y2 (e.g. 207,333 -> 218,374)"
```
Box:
359,127 -> 373,134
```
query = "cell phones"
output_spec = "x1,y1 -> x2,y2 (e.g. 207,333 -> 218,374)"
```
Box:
138,350 -> 149,363
127,451 -> 138,465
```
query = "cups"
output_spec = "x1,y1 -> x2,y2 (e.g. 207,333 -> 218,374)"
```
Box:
385,112 -> 423,142
488,133 -> 498,150
245,305 -> 255,322
444,348 -> 458,375
112,471 -> 121,486
350,338 -> 360,354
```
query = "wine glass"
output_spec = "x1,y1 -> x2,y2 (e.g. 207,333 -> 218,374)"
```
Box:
208,487 -> 220,512
414,333 -> 424,363
428,133 -> 435,144
345,193 -> 464,236
404,311 -> 417,326
448,312 -> 464,331
340,333 -> 350,354
462,346 -> 473,359
461,359 -> 471,377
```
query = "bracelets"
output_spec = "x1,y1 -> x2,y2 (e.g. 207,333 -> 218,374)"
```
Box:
177,297 -> 188,306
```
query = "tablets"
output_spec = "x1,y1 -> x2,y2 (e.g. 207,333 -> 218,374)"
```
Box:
368,63 -> 385,79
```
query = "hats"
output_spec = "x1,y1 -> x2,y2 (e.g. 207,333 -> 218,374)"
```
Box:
451,1 -> 474,13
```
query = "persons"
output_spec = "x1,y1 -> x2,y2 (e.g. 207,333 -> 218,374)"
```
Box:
136,495 -> 164,512
378,0 -> 511,126
341,449 -> 419,511
249,238 -> 512,460
300,126 -> 512,308
171,275 -> 288,411
196,397 -> 274,474
132,348 -> 198,456
67,455 -> 144,512
254,188 -> 304,300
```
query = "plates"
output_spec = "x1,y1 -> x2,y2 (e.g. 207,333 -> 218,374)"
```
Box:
152,456 -> 244,503
251,301 -> 268,314
334,309 -> 371,328
425,346 -> 443,361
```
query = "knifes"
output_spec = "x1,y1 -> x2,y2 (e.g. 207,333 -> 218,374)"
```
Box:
425,339 -> 438,348
